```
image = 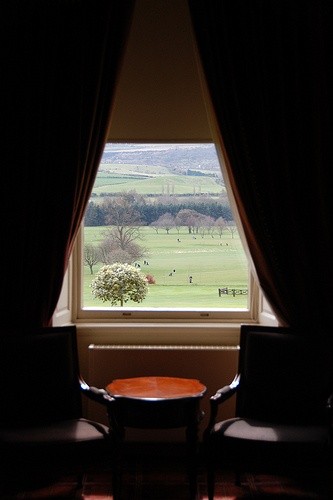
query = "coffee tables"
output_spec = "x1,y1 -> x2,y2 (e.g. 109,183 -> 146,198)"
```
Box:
104,377 -> 207,499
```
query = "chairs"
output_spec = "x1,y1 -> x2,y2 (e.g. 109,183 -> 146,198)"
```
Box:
0,326 -> 115,492
202,326 -> 333,500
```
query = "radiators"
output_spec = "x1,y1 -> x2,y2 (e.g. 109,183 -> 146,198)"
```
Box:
87,345 -> 240,441
